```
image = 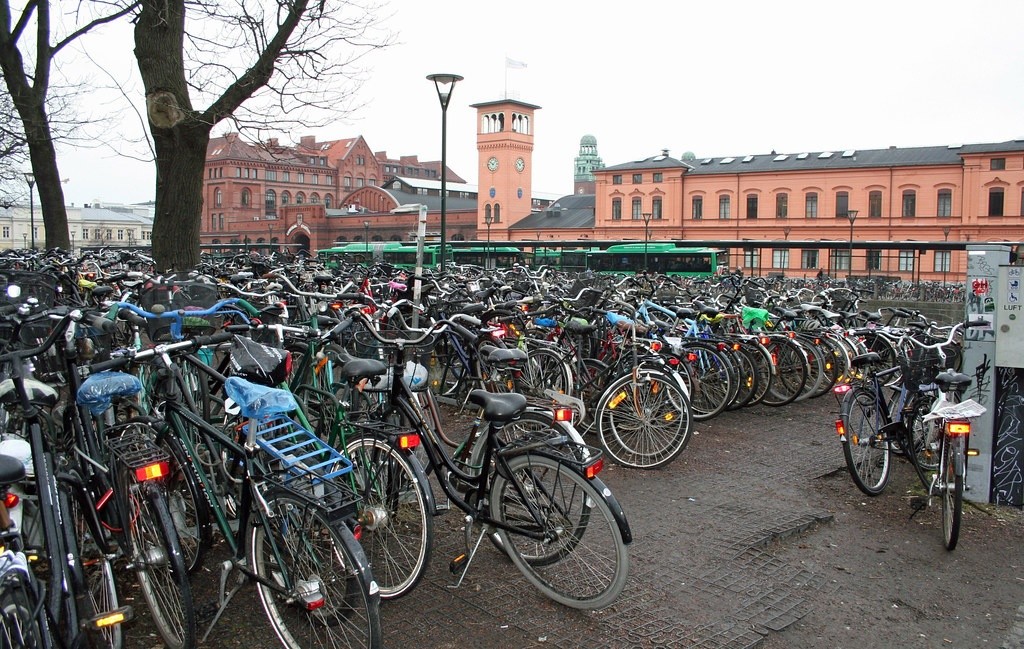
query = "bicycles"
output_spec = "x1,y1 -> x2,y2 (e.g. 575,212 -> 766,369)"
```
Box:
895,318 -> 990,551
0,243 -> 958,649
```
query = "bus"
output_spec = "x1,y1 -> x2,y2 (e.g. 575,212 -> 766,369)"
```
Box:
450,248 -> 534,271
529,243 -> 731,282
315,243 -> 453,274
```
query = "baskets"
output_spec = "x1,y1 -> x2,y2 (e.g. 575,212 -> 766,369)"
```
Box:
567,272 -> 605,308
899,349 -> 958,391
19,322 -> 113,371
0,270 -> 57,337
140,272 -> 218,341
354,329 -> 436,393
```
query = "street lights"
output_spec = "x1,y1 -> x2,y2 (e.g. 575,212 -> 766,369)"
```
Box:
642,214 -> 652,268
941,227 -> 951,287
485,217 -> 494,270
268,223 -> 274,255
846,210 -> 858,275
128,230 -> 131,252
362,220 -> 371,261
23,172 -> 38,254
70,231 -> 76,252
425,71 -> 464,276
